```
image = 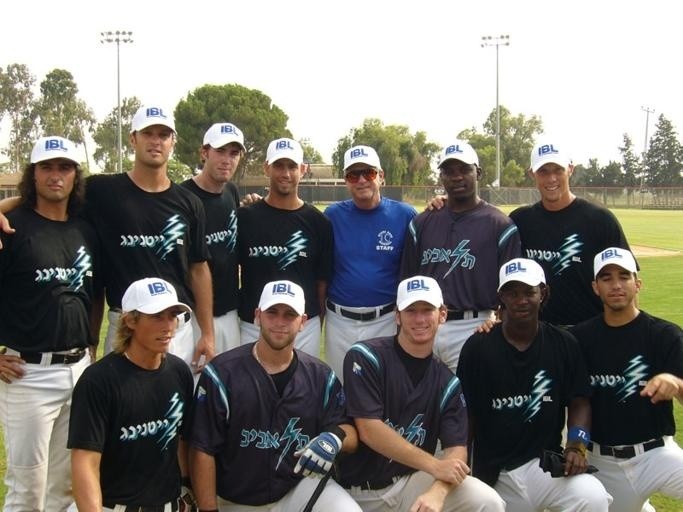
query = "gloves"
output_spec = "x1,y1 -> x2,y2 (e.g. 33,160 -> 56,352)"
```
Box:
293,432 -> 343,480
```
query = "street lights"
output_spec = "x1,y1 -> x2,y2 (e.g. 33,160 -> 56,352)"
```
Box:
477,32 -> 513,185
99,28 -> 136,174
637,102 -> 657,191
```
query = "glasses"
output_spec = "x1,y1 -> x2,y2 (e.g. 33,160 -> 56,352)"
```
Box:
346,169 -> 377,183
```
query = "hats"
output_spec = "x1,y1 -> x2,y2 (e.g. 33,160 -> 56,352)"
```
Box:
437,142 -> 479,168
203,122 -> 246,153
266,137 -> 304,165
593,247 -> 637,279
497,258 -> 546,291
258,279 -> 305,316
397,275 -> 443,311
531,142 -> 570,173
130,106 -> 177,135
343,145 -> 380,170
31,135 -> 82,166
122,277 -> 193,314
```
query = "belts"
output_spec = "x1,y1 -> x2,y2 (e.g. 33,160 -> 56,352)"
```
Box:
104,499 -> 179,511
21,351 -> 87,364
339,480 -> 391,490
446,312 -> 477,320
588,440 -> 662,459
328,304 -> 394,322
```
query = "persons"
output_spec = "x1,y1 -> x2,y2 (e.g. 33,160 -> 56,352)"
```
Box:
190,277 -> 363,512
344,274 -> 509,511
1,103 -> 214,466
425,138 -> 639,336
399,141 -> 520,373
235,138 -> 335,374
68,244 -> 221,510
461,258 -> 617,511
0,136 -> 103,512
571,246 -> 682,510
178,123 -> 245,392
236,144 -> 417,387
